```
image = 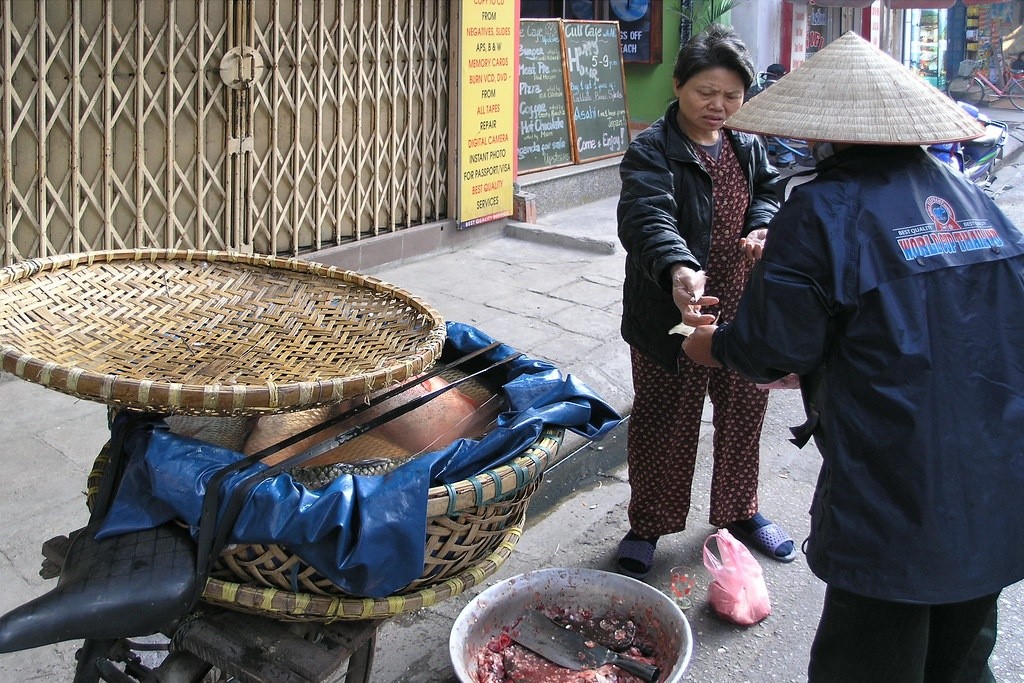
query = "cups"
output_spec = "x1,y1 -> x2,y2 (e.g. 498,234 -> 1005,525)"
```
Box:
671,566 -> 692,609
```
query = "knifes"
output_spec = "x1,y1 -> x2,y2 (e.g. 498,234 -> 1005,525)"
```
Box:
508,611 -> 660,683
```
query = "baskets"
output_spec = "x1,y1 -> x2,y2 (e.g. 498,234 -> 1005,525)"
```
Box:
0,247 -> 447,411
89,440 -> 526,620
107,322 -> 565,598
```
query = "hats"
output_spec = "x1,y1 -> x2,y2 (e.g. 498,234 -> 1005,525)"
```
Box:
722,30 -> 987,145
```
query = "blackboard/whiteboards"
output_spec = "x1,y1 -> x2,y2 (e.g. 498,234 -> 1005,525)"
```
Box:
516,18 -> 632,174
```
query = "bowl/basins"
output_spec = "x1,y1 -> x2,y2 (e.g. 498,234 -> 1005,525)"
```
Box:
449,568 -> 693,683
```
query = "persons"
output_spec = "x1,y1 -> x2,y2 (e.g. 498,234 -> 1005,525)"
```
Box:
617,22 -> 798,578
681,30 -> 1024,683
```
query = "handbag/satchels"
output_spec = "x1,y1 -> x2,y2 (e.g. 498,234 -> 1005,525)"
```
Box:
703,529 -> 771,627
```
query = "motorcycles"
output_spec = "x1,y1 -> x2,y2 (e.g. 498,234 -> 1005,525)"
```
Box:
924,100 -> 1009,193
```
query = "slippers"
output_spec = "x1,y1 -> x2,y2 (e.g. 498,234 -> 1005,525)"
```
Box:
617,541 -> 655,578
731,523 -> 797,562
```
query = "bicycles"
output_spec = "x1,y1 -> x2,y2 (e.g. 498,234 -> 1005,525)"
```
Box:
0,519 -> 378,683
947,60 -> 1024,112
